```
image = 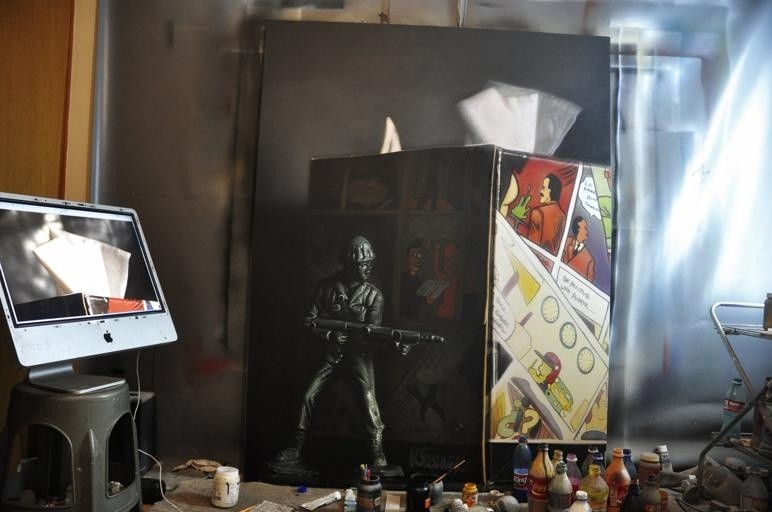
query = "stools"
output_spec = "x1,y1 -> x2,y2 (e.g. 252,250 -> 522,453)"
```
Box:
3,374 -> 145,512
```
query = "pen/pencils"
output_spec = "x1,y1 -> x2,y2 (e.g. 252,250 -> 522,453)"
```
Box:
238,505 -> 256,512
359,463 -> 371,481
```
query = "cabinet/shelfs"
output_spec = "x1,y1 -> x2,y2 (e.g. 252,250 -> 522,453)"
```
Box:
692,293 -> 770,508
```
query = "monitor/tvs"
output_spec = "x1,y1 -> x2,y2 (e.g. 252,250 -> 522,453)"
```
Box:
0,190 -> 178,395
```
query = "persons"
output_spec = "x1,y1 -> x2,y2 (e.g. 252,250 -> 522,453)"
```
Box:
271,236 -> 448,466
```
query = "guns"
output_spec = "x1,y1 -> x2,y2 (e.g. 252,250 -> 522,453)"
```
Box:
311,318 -> 444,356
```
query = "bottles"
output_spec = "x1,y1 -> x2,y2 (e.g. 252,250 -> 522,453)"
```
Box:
430,480 -> 444,506
720,377 -> 746,441
451,499 -> 465,512
405,472 -> 432,512
750,376 -> 772,458
489,489 -> 500,508
356,475 -> 382,512
512,436 -> 532,504
527,440 -> 670,512
210,466 -> 241,508
462,482 -> 478,506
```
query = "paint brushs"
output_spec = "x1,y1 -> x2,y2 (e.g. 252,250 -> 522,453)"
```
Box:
432,459 -> 466,484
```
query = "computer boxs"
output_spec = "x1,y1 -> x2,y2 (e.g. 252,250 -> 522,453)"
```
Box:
109,390 -> 158,477
